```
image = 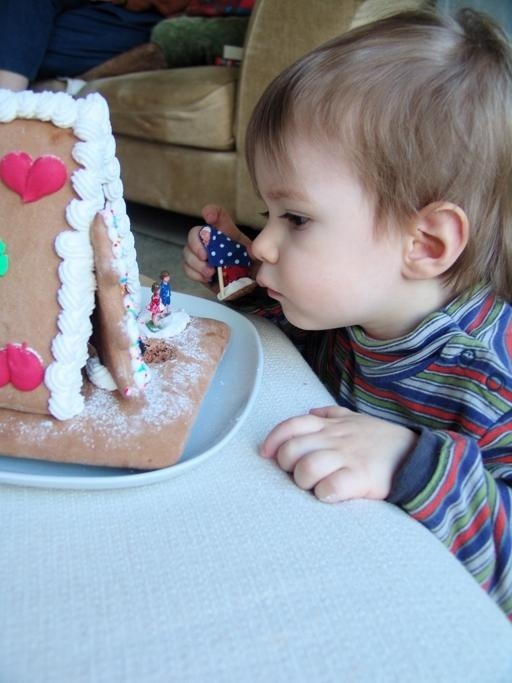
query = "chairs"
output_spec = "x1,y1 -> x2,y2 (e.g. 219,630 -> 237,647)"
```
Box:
46,0 -> 365,216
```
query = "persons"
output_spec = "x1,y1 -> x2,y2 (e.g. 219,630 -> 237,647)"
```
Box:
181,0 -> 512,623
0,0 -> 261,92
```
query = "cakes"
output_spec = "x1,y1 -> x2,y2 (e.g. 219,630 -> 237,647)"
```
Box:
0,88 -> 191,422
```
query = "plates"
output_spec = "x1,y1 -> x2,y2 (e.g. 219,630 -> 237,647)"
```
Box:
1,284 -> 263,487
